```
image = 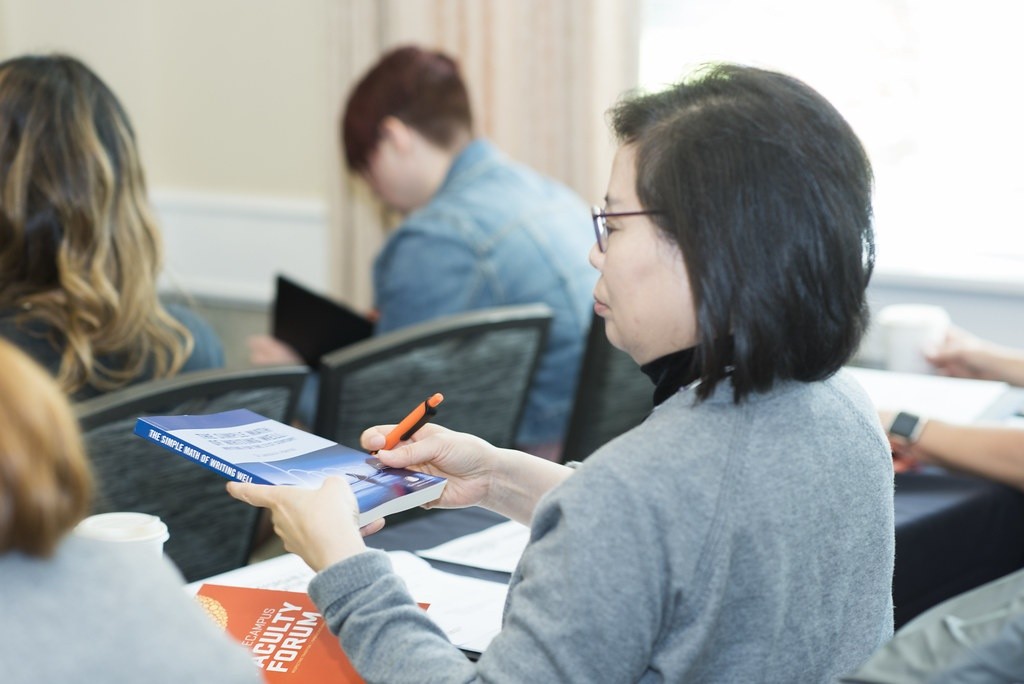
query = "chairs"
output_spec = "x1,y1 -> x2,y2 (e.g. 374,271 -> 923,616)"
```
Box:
314,304 -> 560,453
75,360 -> 311,579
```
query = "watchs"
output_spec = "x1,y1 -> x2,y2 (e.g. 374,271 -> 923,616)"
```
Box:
891,411 -> 928,448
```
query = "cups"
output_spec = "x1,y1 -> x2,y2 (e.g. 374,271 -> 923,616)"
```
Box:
879,304 -> 946,375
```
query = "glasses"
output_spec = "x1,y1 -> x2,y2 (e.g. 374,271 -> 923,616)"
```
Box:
591,206 -> 669,253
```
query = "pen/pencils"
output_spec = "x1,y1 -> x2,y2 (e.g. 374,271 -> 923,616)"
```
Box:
369,393 -> 444,455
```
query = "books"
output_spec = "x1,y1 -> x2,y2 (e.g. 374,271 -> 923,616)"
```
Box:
271,276 -> 379,370
134,407 -> 447,530
187,582 -> 432,684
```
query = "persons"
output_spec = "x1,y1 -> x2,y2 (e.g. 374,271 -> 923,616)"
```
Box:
248,44 -> 604,460
227,65 -> 895,684
872,327 -> 1024,684
0,54 -> 225,404
0,338 -> 262,684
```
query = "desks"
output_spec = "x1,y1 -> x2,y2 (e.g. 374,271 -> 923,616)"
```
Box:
185,462 -> 1024,683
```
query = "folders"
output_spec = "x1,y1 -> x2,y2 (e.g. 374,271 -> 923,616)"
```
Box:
273,276 -> 376,372
